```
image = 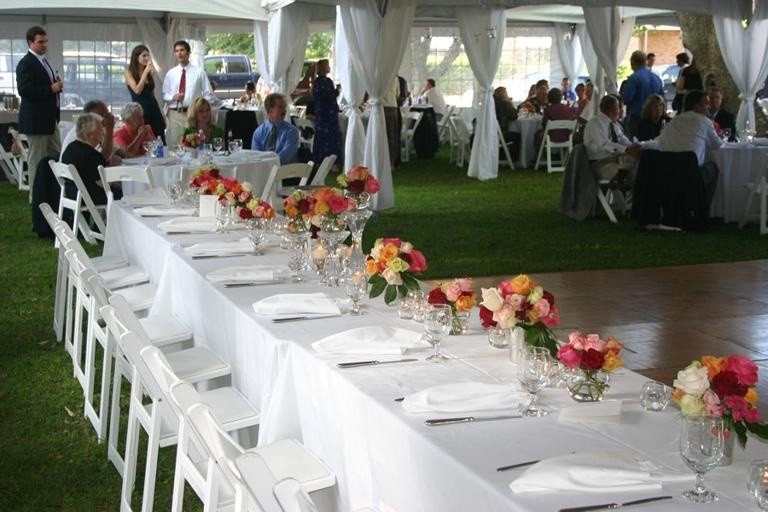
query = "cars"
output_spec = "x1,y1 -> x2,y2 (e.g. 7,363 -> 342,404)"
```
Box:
290,58 -> 319,102
649,64 -> 680,104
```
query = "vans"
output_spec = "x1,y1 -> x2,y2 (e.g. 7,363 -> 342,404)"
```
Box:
0,53 -> 25,106
59,58 -> 134,110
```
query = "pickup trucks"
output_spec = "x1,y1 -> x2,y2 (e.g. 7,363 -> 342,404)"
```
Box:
203,54 -> 260,100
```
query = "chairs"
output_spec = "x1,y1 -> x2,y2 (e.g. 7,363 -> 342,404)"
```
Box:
1,96 -> 766,511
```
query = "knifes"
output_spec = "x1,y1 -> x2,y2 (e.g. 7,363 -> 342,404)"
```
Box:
191,252 -> 246,259
166,231 -> 215,235
222,281 -> 292,291
271,315 -> 344,321
424,414 -> 522,425
557,496 -> 674,511
336,357 -> 418,369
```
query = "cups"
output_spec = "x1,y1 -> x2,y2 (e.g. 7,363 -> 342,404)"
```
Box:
557,361 -> 575,382
397,291 -> 429,323
747,458 -> 768,511
266,214 -> 350,280
639,381 -> 669,411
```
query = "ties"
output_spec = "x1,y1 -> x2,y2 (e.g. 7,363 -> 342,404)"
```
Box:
609,121 -> 619,145
43,55 -> 57,88
268,126 -> 277,152
178,69 -> 187,96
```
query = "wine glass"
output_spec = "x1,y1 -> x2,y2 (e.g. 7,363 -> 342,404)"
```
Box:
423,303 -> 453,362
215,200 -> 230,236
680,413 -> 726,505
516,347 -> 554,420
167,181 -> 206,207
141,130 -> 245,165
344,267 -> 369,317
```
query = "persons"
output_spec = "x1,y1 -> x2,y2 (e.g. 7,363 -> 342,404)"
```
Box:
16,26 -> 63,205
163,40 -> 215,151
0,122 -> 29,181
58,100 -> 126,165
179,98 -> 225,150
383,73 -> 447,169
124,44 -> 167,147
113,102 -> 157,159
61,111 -> 123,243
240,59 -> 343,173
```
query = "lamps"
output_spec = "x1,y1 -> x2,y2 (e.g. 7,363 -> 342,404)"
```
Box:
417,21 -> 497,45
